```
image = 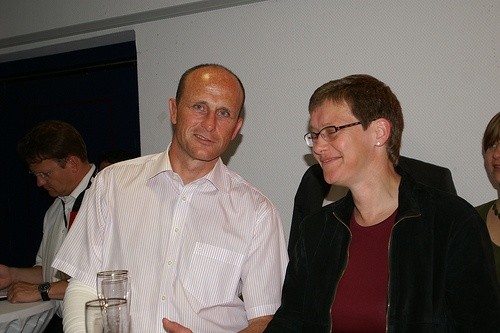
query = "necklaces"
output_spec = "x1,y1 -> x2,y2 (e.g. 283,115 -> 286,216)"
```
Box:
491,203 -> 500,221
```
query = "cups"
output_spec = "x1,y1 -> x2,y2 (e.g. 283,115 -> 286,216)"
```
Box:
85,298 -> 128,333
96,270 -> 132,333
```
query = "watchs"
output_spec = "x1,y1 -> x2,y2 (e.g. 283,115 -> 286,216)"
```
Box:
38,281 -> 52,302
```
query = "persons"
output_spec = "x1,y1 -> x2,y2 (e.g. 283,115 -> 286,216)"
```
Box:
50,63 -> 290,333
472,111 -> 500,333
0,127 -> 107,333
258,74 -> 483,333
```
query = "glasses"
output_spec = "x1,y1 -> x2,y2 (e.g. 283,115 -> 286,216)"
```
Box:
304,119 -> 375,147
29,166 -> 59,180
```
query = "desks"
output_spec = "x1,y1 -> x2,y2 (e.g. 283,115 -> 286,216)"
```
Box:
0,299 -> 58,333
